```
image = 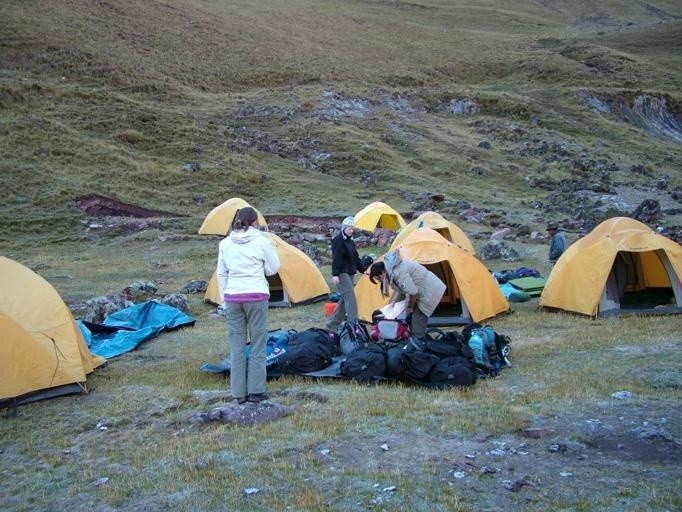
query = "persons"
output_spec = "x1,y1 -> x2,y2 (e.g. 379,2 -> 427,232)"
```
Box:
545,222 -> 567,266
216,207 -> 281,404
325,216 -> 364,327
369,251 -> 447,337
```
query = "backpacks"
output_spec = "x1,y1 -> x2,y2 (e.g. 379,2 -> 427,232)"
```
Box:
338,326 -> 475,387
266,323 -> 335,376
469,325 -> 510,375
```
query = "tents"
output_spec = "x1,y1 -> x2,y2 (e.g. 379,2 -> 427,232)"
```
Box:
203,233 -> 331,306
354,228 -> 509,324
353,202 -> 407,231
389,211 -> 476,256
198,198 -> 269,234
537,216 -> 682,318
0,255 -> 107,403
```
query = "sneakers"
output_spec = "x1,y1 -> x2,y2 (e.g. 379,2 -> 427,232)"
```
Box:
248,394 -> 269,402
238,397 -> 246,404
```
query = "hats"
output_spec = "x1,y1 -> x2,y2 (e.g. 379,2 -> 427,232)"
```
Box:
545,223 -> 559,231
340,216 -> 355,240
